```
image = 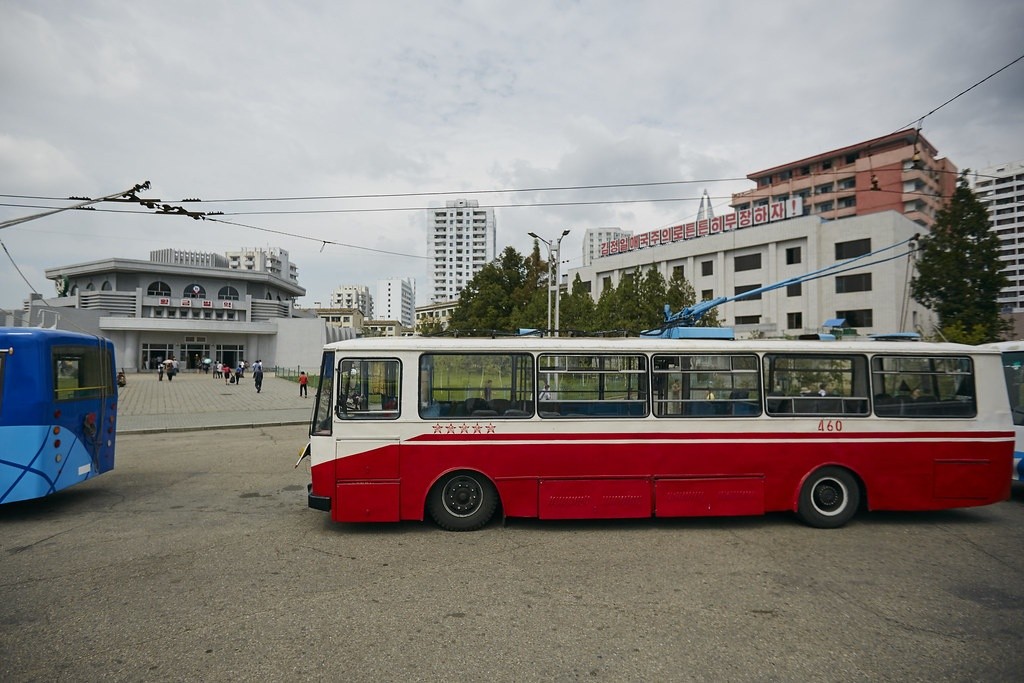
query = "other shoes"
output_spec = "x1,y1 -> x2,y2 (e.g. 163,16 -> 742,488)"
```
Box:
305,395 -> 307,398
257,388 -> 260,392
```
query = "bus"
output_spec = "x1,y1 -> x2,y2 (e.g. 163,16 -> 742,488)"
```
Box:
0,180 -> 157,507
291,233 -> 1023,533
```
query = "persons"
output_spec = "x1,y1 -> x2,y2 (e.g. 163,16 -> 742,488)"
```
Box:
252,359 -> 264,393
672,379 -> 681,408
729,381 -> 750,399
539,385 -> 552,400
213,360 -> 248,386
706,385 -> 715,400
195,355 -> 209,374
484,380 -> 496,401
298,371 -> 309,398
143,354 -> 179,381
818,384 -> 827,397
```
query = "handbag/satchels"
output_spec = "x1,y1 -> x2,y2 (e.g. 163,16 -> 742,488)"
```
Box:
230,374 -> 235,382
235,371 -> 239,375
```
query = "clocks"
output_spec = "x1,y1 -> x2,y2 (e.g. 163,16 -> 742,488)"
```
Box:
55,275 -> 69,294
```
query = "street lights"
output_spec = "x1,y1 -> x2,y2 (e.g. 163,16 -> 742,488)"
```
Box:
527,229 -> 571,404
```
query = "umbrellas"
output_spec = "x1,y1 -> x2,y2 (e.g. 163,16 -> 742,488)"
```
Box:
162,359 -> 173,363
204,358 -> 212,364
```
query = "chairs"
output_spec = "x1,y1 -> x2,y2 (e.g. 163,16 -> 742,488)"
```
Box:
422,398 -> 977,417
